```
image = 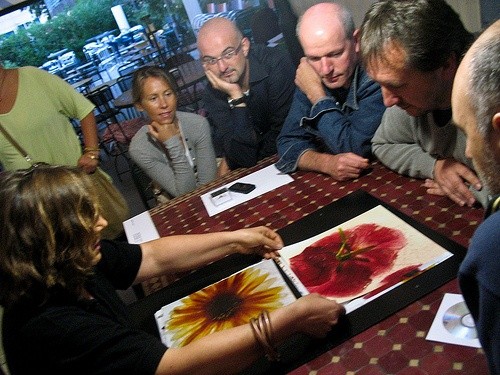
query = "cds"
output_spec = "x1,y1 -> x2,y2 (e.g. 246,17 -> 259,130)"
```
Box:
442,301 -> 478,340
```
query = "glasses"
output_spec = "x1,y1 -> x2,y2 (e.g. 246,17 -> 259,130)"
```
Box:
200,42 -> 242,66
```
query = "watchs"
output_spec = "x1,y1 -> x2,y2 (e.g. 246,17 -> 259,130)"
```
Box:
226,94 -> 248,110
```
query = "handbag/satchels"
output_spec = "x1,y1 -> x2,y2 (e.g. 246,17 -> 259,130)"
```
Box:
147,158 -> 230,204
67,165 -> 131,241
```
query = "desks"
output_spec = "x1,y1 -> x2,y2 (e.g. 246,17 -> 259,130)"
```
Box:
114,61 -> 207,110
140,136 -> 489,375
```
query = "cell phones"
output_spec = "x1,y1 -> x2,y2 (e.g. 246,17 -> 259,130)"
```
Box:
229,182 -> 255,194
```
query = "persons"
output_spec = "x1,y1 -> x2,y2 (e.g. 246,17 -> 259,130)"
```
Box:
1,163 -> 348,373
0,62 -> 114,189
126,64 -> 218,208
273,2 -> 387,183
450,17 -> 500,375
196,17 -> 299,170
358,0 -> 496,211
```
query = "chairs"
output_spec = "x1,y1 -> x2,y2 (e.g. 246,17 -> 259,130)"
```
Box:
41,45 -> 216,160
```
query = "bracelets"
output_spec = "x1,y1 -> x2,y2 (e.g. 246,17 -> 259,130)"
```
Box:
249,309 -> 281,362
